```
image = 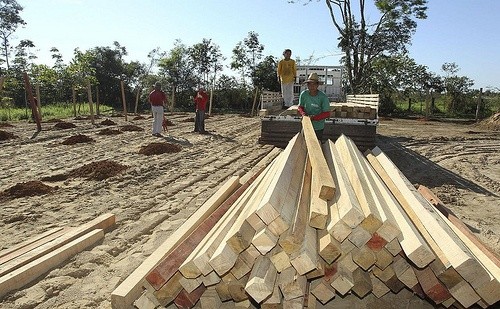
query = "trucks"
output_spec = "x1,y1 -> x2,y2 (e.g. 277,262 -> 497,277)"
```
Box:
258,65 -> 380,149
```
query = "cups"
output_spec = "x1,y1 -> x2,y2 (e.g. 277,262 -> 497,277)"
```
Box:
190,96 -> 193,100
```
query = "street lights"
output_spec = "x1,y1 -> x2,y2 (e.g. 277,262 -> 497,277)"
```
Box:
247,49 -> 257,88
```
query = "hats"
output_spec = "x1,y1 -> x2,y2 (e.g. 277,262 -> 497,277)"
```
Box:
303,73 -> 324,86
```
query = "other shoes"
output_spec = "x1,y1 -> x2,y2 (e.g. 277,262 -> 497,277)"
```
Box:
282,106 -> 290,110
152,133 -> 163,137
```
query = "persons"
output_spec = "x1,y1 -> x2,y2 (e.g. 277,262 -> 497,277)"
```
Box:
150,81 -> 169,137
298,72 -> 331,143
194,87 -> 209,132
277,49 -> 297,109
